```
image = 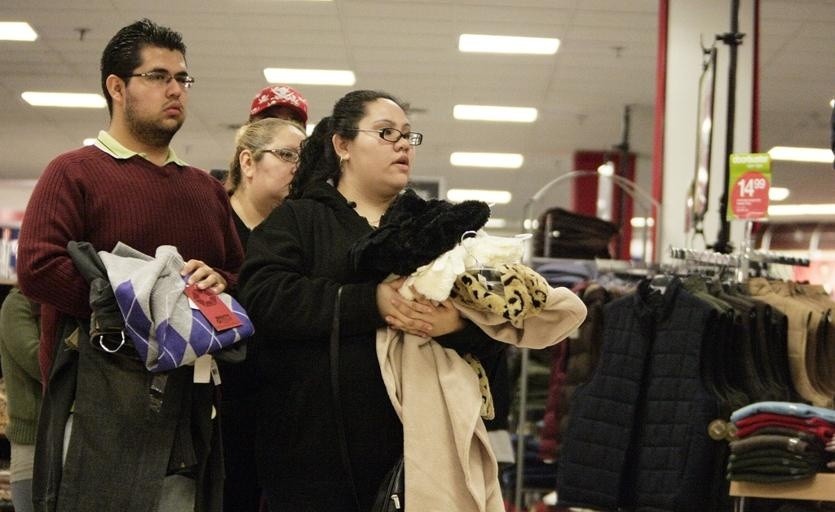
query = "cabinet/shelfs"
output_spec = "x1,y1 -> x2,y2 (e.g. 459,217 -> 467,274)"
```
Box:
504,249 -> 629,512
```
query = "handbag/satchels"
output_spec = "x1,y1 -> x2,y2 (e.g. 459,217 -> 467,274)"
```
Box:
369,457 -> 404,512
534,208 -> 619,259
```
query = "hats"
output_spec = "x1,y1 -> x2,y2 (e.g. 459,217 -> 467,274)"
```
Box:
250,85 -> 308,122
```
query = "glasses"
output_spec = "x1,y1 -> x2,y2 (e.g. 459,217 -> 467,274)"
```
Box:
261,148 -> 301,163
354,127 -> 423,145
131,69 -> 195,87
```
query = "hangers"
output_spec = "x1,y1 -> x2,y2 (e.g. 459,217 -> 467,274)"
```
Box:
581,247 -> 814,304
